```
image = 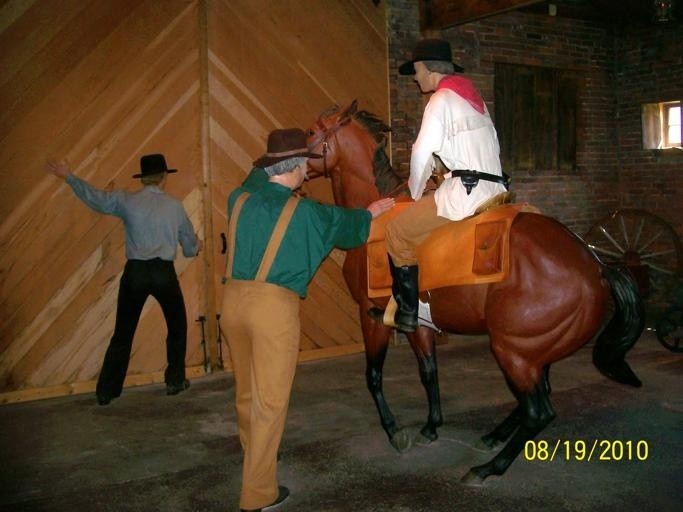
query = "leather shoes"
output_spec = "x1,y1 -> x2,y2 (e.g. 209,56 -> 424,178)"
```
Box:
166,379 -> 190,396
239,453 -> 290,512
97,393 -> 120,406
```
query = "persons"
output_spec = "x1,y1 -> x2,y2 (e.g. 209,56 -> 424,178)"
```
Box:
363,37 -> 507,336
216,122 -> 399,512
43,151 -> 204,407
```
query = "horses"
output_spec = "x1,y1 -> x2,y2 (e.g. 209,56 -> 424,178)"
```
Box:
304,98 -> 644,487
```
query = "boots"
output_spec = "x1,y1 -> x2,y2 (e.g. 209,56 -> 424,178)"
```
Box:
366,252 -> 419,333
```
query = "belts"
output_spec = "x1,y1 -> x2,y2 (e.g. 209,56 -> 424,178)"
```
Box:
452,169 -> 511,184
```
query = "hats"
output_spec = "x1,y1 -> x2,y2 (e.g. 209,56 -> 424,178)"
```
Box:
253,129 -> 322,169
132,154 -> 178,179
399,39 -> 465,76
